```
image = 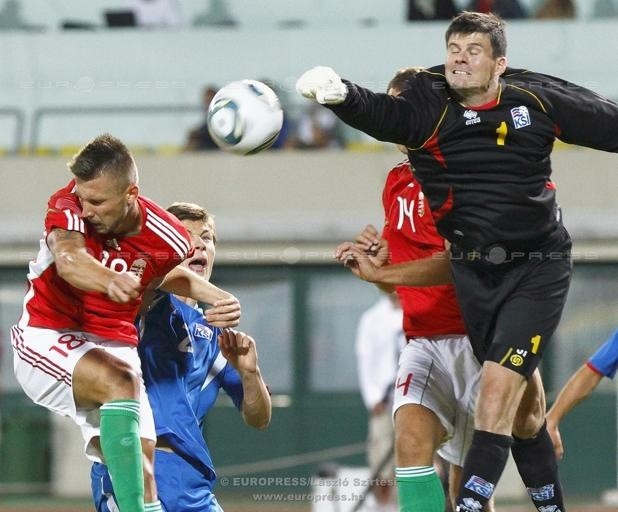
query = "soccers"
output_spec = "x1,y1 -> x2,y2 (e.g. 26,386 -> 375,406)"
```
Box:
206,80 -> 283,155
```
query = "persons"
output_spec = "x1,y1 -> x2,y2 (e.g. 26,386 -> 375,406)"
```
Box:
543,327 -> 618,461
334,66 -> 495,511
353,279 -> 457,511
178,81 -> 368,154
10,132 -> 193,511
127,0 -> 178,24
90,200 -> 271,511
402,0 -> 618,23
294,10 -> 618,511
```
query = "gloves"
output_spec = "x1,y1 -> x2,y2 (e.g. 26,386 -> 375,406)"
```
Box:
297,66 -> 348,104
